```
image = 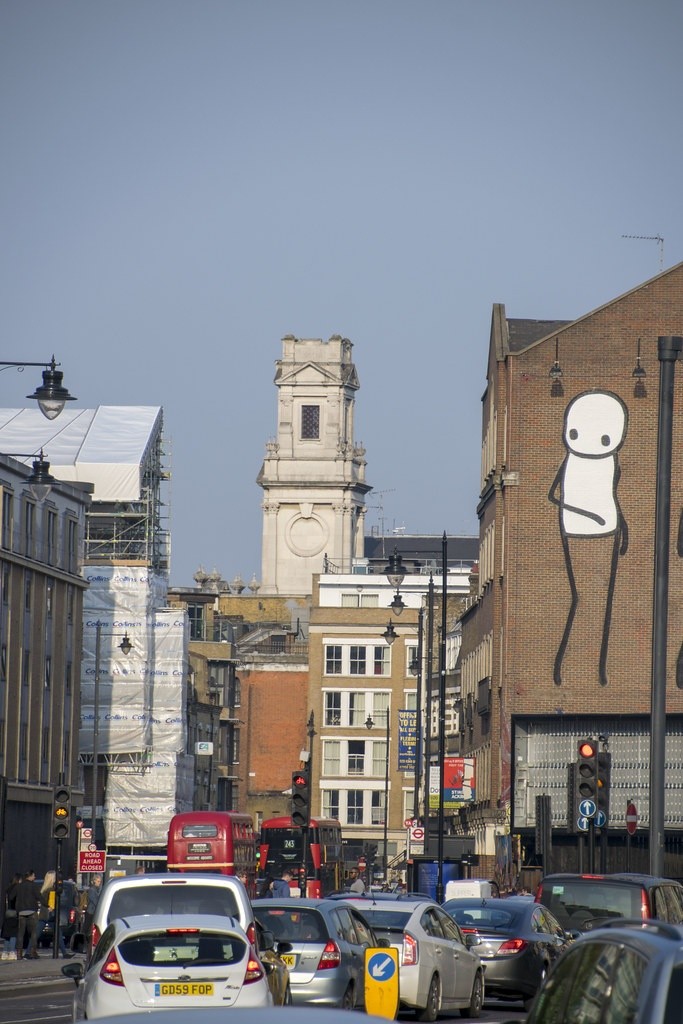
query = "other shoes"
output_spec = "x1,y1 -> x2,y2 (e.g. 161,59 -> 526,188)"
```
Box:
64,954 -> 76,958
24,954 -> 32,959
17,950 -> 24,960
30,949 -> 40,958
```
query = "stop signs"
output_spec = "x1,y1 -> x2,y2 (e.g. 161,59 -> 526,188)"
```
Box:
624,804 -> 637,835
357,859 -> 367,872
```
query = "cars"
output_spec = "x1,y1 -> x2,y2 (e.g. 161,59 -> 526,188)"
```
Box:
441,897 -> 582,1006
538,872 -> 683,940
61,913 -> 274,1023
251,899 -> 390,1010
339,899 -> 487,1019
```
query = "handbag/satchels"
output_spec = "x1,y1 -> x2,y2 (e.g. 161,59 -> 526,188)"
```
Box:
47,891 -> 55,908
6,910 -> 17,917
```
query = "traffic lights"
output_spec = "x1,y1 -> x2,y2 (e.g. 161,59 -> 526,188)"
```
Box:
595,753 -> 611,827
52,785 -> 72,840
368,844 -> 378,862
291,772 -> 311,827
576,739 -> 598,818
57,878 -> 63,894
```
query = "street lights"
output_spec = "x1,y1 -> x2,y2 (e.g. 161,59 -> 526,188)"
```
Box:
91,631 -> 136,846
366,714 -> 388,880
381,621 -> 430,855
388,589 -> 434,857
381,556 -> 447,897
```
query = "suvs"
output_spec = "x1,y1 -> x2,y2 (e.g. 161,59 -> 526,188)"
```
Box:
504,917 -> 683,1024
324,889 -> 438,924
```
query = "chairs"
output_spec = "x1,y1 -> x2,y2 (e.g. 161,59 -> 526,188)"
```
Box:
134,899 -> 157,915
263,916 -> 321,937
199,899 -> 228,915
555,892 -> 632,923
461,912 -> 508,927
195,939 -> 225,961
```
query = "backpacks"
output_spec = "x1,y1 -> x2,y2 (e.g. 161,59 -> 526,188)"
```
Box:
79,891 -> 89,907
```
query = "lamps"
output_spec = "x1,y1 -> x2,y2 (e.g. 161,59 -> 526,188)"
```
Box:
632,335 -> 646,378
549,338 -> 563,378
452,698 -> 466,713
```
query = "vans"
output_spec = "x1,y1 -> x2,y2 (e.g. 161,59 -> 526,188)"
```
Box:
70,872 -> 274,963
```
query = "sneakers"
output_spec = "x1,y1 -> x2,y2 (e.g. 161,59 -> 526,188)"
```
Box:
1,953 -> 8,960
8,952 -> 17,960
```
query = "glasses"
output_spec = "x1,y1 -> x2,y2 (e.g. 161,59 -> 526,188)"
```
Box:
350,871 -> 356,874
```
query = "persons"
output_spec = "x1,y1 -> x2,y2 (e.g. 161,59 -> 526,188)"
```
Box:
236,870 -> 248,889
345,867 -> 365,893
394,879 -> 406,894
259,877 -> 274,898
24,870 -> 75,959
505,885 -> 517,896
522,887 -> 531,896
9,870 -> 48,960
273,869 -> 293,898
83,874 -> 102,932
381,882 -> 392,893
499,886 -> 508,899
1,872 -> 22,959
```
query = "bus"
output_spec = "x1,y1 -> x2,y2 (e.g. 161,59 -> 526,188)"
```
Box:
167,810 -> 260,899
344,844 -> 369,891
258,816 -> 346,923
